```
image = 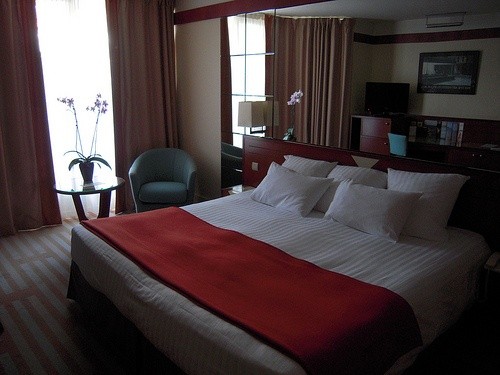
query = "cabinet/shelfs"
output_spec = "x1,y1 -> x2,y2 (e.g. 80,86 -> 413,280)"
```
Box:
348,116 -> 408,155
405,138 -> 500,173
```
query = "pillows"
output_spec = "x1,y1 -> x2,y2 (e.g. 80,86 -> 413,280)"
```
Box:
252,162 -> 333,217
383,168 -> 468,244
323,183 -> 421,241
313,165 -> 387,212
281,155 -> 338,177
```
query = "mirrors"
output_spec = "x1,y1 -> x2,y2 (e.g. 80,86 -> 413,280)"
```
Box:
219,0 -> 500,197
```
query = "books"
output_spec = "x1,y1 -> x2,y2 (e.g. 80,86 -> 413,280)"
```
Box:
409,120 -> 464,148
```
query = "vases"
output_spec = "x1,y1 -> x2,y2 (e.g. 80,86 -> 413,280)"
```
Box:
79,163 -> 93,183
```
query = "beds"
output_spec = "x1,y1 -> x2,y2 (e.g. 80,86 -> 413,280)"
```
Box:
67,135 -> 488,375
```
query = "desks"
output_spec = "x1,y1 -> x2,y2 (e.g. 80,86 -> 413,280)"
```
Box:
55,174 -> 123,222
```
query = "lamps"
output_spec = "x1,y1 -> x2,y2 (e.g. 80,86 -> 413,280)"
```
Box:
238,101 -> 262,134
263,101 -> 279,133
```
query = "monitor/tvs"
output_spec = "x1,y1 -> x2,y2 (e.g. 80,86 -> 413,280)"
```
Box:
364,81 -> 411,118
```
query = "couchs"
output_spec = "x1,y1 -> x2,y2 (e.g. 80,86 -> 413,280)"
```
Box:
130,148 -> 197,213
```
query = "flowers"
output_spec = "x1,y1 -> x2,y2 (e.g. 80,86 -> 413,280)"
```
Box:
57,94 -> 112,170
287,91 -> 303,135
